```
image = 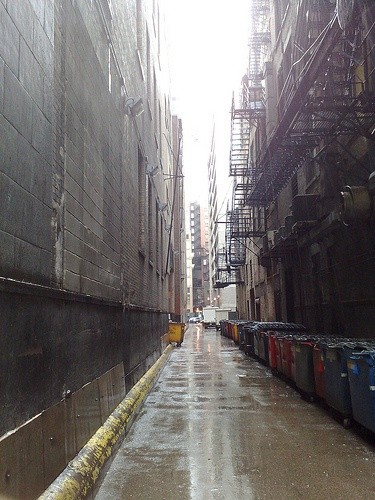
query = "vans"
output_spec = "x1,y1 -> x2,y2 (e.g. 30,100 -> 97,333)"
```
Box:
189,317 -> 201,324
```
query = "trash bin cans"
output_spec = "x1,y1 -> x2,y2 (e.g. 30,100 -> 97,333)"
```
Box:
168,321 -> 186,347
219,318 -> 375,434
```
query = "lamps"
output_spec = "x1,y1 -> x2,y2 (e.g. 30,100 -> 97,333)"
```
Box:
124,97 -> 145,120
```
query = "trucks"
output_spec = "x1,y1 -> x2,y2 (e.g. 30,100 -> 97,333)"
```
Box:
202,305 -> 219,329
215,308 -> 232,331
